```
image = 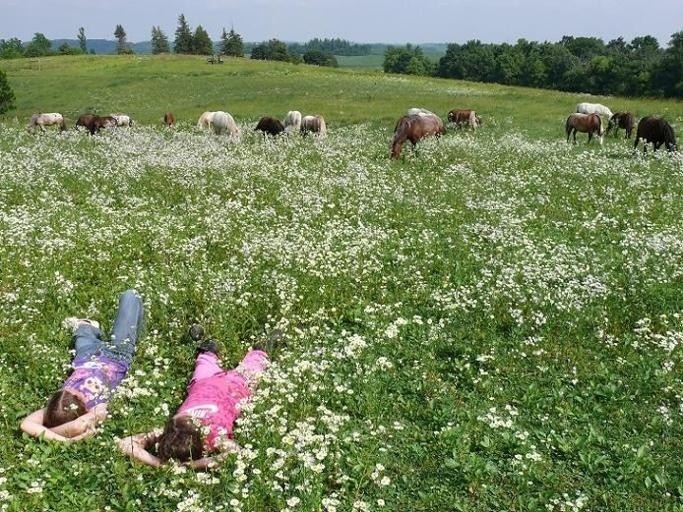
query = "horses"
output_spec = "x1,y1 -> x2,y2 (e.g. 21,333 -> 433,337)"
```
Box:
280,111 -> 301,130
300,115 -> 326,137
633,116 -> 678,155
566,103 -> 634,144
164,112 -> 174,126
253,117 -> 285,136
389,106 -> 445,159
76,113 -> 134,136
29,113 -> 64,132
197,111 -> 241,137
448,108 -> 480,130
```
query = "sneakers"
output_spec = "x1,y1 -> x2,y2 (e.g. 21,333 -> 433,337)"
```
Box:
62,316 -> 99,333
254,330 -> 282,350
189,324 -> 219,354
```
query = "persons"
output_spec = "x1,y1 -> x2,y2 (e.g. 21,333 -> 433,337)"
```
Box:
19,288 -> 145,448
116,324 -> 284,474
